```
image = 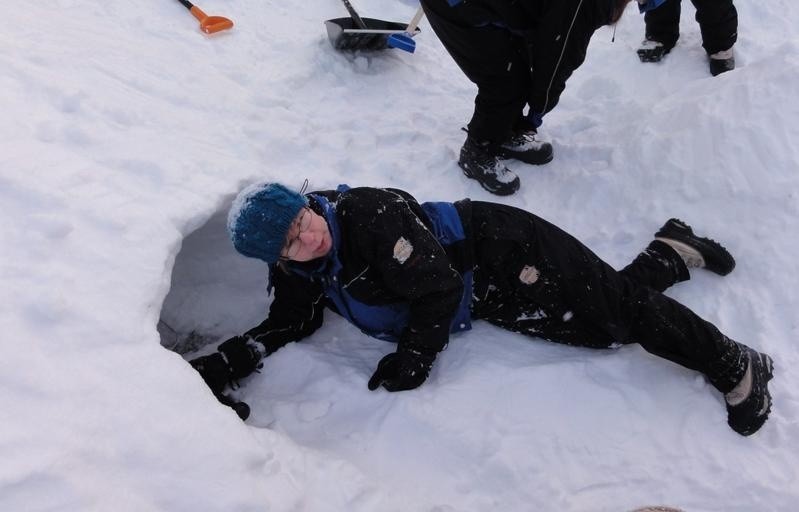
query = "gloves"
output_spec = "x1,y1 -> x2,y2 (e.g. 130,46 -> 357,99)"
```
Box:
186,334 -> 257,391
364,342 -> 438,393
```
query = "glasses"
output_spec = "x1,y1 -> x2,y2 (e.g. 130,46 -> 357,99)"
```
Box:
279,206 -> 313,260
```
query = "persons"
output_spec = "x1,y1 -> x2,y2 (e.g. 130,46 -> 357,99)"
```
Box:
183,178 -> 775,438
419,2 -> 632,198
632,1 -> 740,78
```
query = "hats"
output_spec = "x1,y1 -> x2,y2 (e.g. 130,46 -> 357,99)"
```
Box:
229,179 -> 309,264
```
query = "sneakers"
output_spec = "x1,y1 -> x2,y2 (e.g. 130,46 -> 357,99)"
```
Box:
492,130 -> 556,165
709,46 -> 734,77
654,218 -> 735,276
637,38 -> 670,62
725,343 -> 773,437
458,142 -> 521,196
